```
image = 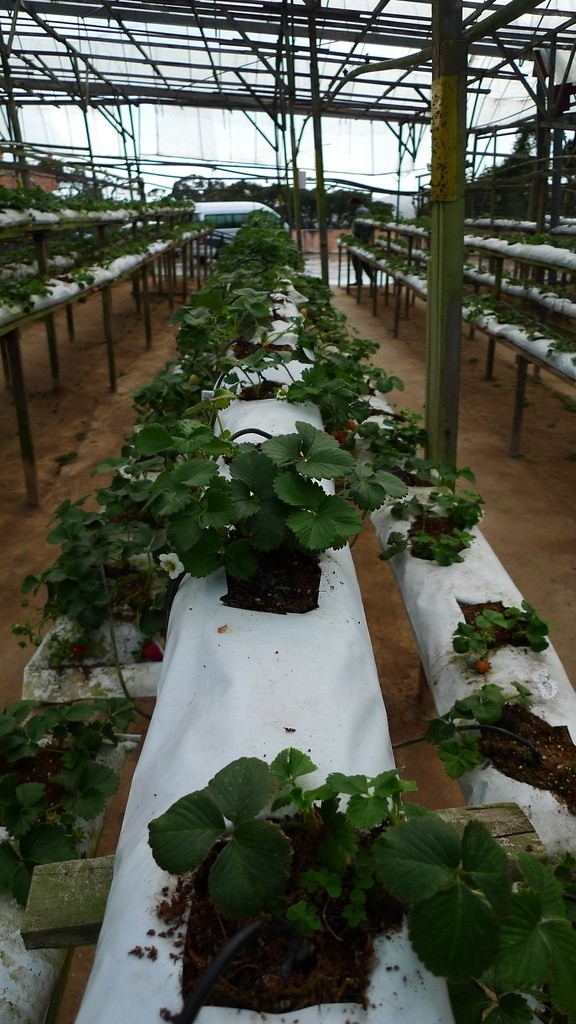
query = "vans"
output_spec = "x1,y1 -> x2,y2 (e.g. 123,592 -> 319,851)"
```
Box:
180,201 -> 291,265
